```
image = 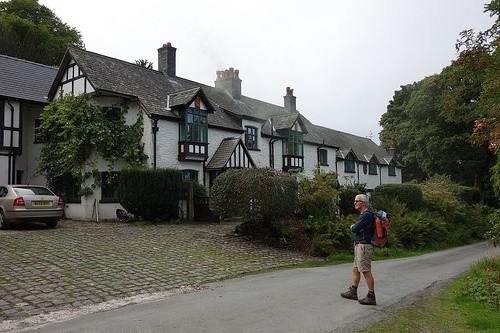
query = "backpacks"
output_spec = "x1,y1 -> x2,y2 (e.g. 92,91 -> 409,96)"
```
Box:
364,208 -> 389,248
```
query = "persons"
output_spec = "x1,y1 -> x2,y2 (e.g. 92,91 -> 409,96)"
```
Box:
341,194 -> 376,305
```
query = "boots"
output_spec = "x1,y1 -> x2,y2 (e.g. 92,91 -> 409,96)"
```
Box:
340,285 -> 358,300
358,291 -> 377,305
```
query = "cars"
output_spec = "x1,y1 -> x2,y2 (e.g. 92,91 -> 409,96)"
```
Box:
0,184 -> 64,230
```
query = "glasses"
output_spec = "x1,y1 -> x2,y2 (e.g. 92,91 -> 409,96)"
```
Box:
354,199 -> 365,203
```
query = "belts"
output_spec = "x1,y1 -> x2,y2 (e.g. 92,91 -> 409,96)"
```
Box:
354,240 -> 373,246
